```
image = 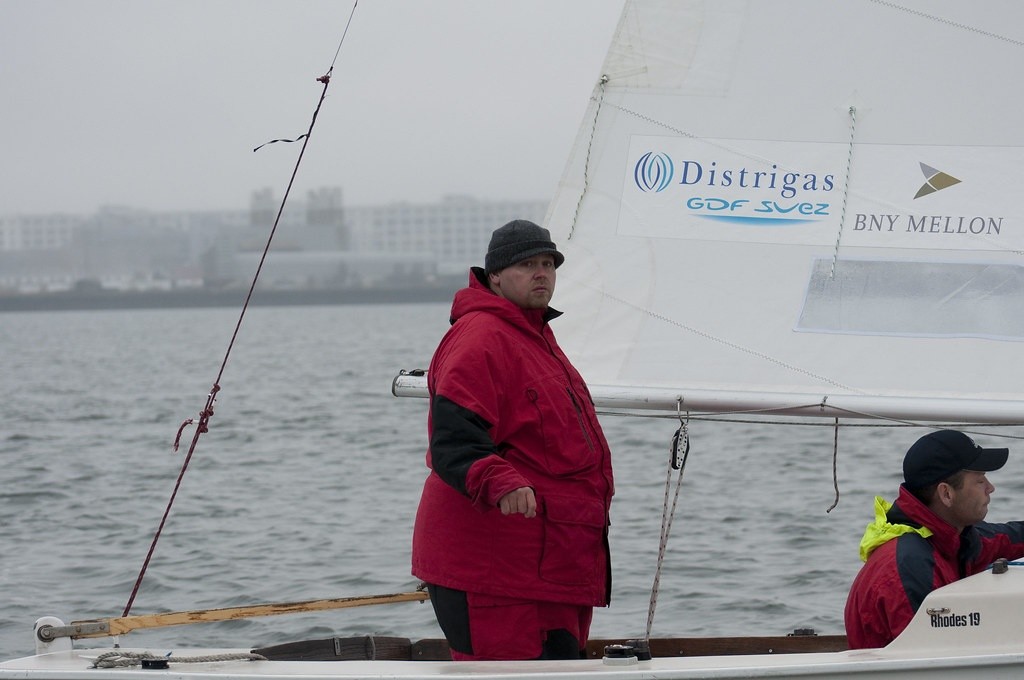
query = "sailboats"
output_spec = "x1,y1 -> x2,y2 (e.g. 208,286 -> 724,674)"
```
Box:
0,0 -> 1024,680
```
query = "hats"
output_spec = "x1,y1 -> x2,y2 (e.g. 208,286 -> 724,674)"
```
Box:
484,219 -> 564,284
902,430 -> 1009,490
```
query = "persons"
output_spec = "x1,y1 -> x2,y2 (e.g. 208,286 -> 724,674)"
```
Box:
844,430 -> 1024,651
410,219 -> 615,661
